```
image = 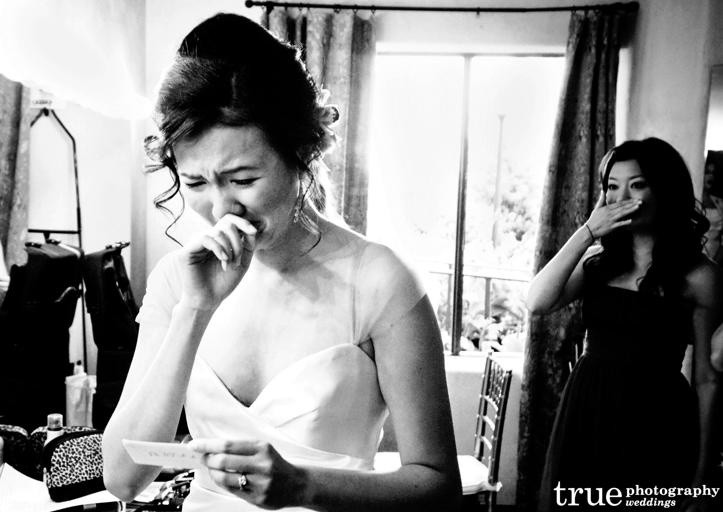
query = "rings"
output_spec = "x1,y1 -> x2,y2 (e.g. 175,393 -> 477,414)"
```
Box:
239,472 -> 247,491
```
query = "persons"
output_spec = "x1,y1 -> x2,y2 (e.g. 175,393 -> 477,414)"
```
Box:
102,14 -> 462,512
526,138 -> 720,512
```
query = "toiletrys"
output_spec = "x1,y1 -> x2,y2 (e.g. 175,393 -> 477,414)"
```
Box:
43,413 -> 63,486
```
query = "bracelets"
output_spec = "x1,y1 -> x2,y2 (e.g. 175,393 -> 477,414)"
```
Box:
585,223 -> 595,241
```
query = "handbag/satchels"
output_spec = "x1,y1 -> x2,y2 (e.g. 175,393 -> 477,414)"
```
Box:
43,430 -> 107,502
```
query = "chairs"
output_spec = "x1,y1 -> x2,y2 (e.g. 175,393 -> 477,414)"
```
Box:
372,350 -> 513,512
